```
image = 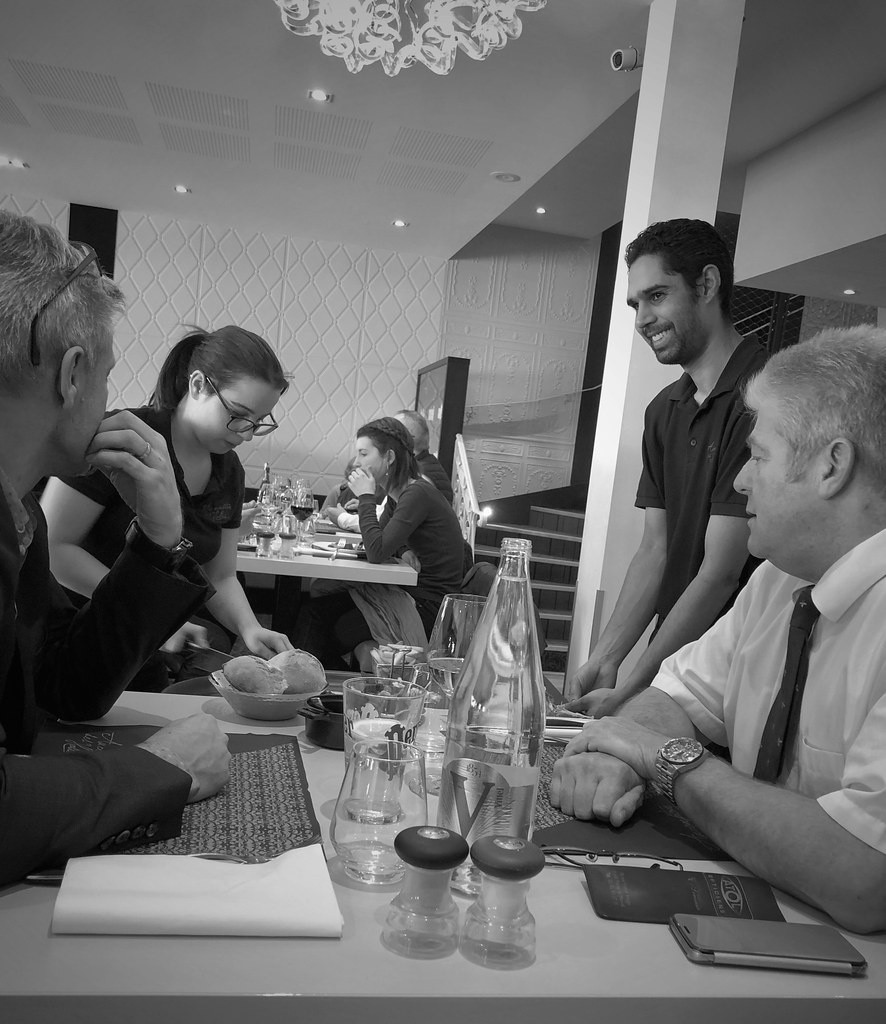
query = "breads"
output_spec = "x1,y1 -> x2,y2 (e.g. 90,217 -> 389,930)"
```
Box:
221,649 -> 328,695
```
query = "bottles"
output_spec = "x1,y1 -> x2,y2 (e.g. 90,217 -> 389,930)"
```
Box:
437,537 -> 548,894
256,532 -> 275,558
385,826 -> 470,958
278,533 -> 296,560
459,835 -> 546,970
258,467 -> 271,490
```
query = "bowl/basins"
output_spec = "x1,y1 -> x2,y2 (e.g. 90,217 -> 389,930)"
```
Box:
208,669 -> 330,721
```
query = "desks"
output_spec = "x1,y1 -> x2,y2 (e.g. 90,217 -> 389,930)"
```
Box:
236,517 -> 417,647
1,691 -> 885,1024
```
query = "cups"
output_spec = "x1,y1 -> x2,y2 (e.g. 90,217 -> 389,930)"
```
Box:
410,663 -> 450,753
329,739 -> 428,884
343,677 -> 427,825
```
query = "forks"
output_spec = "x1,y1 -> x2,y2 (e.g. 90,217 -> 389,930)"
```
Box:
329,537 -> 346,561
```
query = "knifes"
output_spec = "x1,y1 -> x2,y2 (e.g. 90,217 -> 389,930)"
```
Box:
185,640 -> 233,673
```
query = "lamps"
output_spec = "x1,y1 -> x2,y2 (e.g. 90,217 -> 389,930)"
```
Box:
275,0 -> 549,75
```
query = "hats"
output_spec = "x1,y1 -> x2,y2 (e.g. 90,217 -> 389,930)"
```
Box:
367,416 -> 415,454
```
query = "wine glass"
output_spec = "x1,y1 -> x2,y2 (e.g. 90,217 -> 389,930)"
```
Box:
427,594 -> 488,797
291,490 -> 314,551
257,477 -> 319,549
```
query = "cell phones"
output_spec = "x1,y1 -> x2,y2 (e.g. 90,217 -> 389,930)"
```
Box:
669,913 -> 869,977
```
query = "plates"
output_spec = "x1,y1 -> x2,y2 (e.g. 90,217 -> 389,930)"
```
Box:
312,541 -> 368,553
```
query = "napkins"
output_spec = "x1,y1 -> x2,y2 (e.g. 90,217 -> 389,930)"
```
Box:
491,717 -> 593,744
51,844 -> 344,939
293,546 -> 359,559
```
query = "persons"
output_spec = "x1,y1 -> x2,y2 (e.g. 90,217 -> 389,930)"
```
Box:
557,219 -> 776,718
550,324 -> 886,936
35,325 -> 295,690
0,210 -> 232,889
283,411 -> 464,670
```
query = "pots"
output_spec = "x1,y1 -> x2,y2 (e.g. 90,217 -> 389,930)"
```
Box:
296,694 -> 344,750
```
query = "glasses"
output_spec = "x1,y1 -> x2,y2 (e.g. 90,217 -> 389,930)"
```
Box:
30,240 -> 103,366
206,376 -> 278,436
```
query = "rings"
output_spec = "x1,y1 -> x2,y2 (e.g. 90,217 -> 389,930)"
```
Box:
140,442 -> 151,460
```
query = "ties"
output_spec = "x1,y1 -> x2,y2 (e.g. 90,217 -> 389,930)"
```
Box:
754,586 -> 821,786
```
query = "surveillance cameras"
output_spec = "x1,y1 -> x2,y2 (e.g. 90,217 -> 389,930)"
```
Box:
611,47 -> 643,71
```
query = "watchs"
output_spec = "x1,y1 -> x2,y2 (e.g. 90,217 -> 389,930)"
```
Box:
125,516 -> 193,570
655,735 -> 713,808
